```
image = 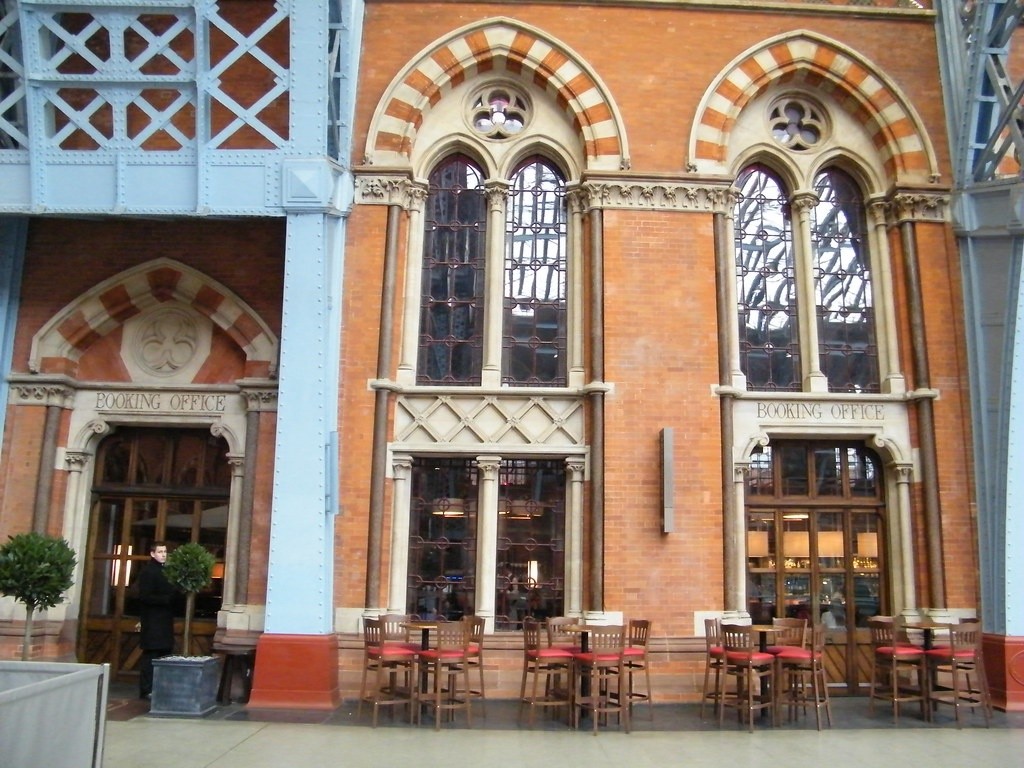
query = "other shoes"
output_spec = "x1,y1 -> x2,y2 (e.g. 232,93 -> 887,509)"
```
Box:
140,691 -> 152,700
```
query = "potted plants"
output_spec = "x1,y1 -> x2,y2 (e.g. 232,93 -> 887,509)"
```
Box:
150,543 -> 226,717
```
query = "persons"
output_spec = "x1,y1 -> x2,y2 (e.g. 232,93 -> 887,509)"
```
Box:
129,542 -> 181,702
502,564 -> 518,593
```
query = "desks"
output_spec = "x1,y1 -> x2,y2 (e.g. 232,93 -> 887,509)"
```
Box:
401,622 -> 446,727
902,623 -> 955,723
752,625 -> 788,727
559,624 -> 602,729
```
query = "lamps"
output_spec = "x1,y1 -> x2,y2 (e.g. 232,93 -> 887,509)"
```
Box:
527,561 -> 538,589
111,544 -> 133,587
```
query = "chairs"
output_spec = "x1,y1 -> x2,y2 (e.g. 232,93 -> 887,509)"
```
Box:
359,613 -> 992,733
